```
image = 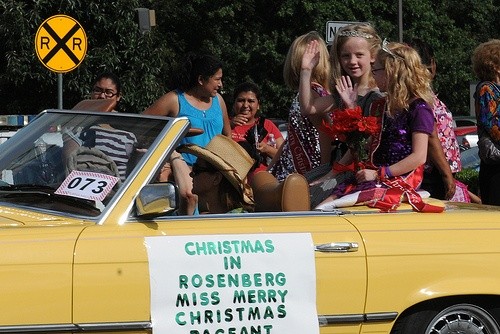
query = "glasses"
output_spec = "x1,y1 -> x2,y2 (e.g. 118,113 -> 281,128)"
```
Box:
192,163 -> 210,176
92,87 -> 116,98
371,68 -> 385,75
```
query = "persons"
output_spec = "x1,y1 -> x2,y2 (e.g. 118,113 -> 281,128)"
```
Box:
128,55 -> 232,184
62,72 -> 139,198
176,134 -> 254,214
473,39 -> 500,207
229,82 -> 285,186
266,22 -> 481,210
169,150 -> 199,216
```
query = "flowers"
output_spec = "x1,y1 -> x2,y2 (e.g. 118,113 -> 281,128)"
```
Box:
320,105 -> 382,170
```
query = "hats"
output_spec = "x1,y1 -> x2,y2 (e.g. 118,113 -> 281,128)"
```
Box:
176,135 -> 255,204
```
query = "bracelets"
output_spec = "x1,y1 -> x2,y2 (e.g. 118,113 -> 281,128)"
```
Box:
378,165 -> 381,180
386,166 -> 392,178
381,165 -> 386,182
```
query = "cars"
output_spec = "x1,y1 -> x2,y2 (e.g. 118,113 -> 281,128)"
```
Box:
452,115 -> 479,152
267,117 -> 289,147
0,114 -> 64,150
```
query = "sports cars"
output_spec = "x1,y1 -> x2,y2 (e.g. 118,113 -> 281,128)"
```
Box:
0,108 -> 500,334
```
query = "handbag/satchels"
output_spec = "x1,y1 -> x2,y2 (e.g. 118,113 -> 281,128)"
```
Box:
477,81 -> 500,167
